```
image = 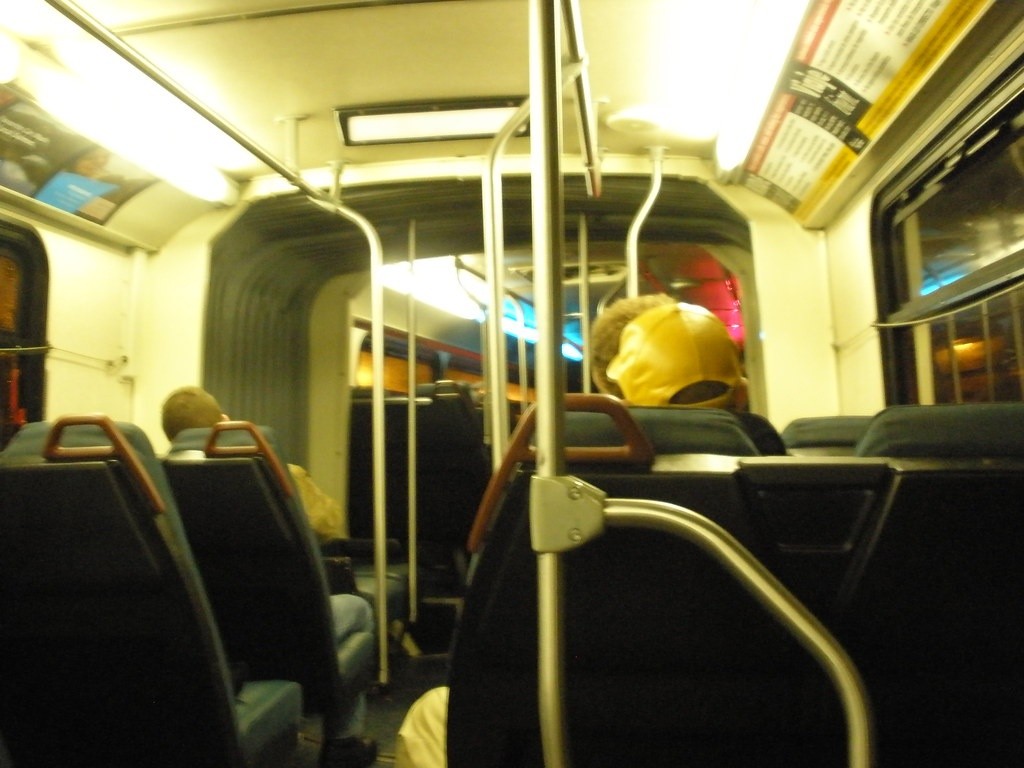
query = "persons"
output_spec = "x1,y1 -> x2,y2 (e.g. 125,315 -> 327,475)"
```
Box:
161,387 -> 378,768
398,292 -> 787,768
0,352 -> 29,453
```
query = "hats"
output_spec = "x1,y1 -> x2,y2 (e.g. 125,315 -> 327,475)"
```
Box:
606,302 -> 750,417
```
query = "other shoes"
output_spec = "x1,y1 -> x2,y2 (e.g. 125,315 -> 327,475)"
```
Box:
319,735 -> 378,768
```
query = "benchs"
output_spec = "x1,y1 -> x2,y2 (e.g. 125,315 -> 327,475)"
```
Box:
2,383 -> 1024,768
